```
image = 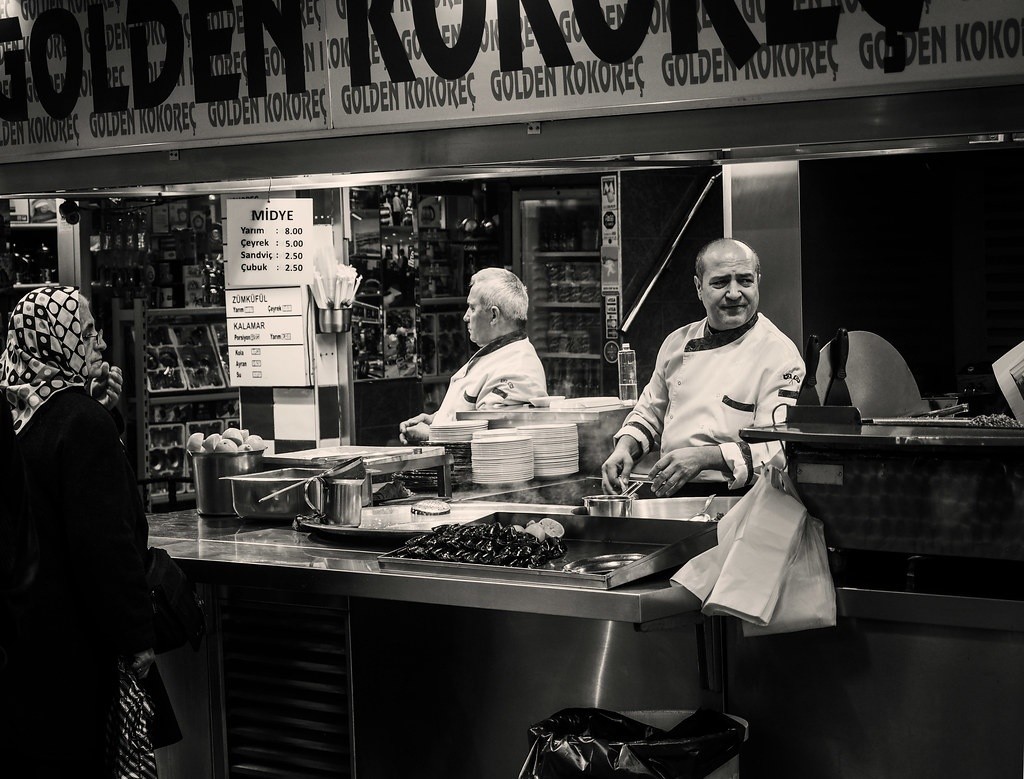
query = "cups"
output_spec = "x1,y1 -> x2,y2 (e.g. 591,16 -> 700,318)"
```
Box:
583,491 -> 633,518
303,475 -> 366,529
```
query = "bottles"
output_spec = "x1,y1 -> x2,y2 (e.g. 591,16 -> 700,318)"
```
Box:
617,342 -> 639,405
348,189 -> 461,300
9,199 -> 220,306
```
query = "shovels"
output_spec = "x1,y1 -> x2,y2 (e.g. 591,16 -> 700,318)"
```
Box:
823,328 -> 853,406
796,334 -> 822,406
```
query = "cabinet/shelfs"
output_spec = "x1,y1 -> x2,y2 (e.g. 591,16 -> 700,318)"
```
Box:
513,189 -> 608,400
112,297 -> 241,513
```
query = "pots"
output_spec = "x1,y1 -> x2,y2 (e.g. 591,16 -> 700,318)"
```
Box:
187,445 -> 267,520
314,306 -> 353,334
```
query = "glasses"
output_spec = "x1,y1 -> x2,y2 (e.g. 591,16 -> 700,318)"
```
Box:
83,328 -> 104,344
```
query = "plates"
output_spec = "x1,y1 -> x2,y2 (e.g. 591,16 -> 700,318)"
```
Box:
404,419 -> 582,494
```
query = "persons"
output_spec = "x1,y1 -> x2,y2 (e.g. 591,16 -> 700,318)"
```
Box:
1,284 -> 202,777
396,266 -> 551,445
601,237 -> 807,498
